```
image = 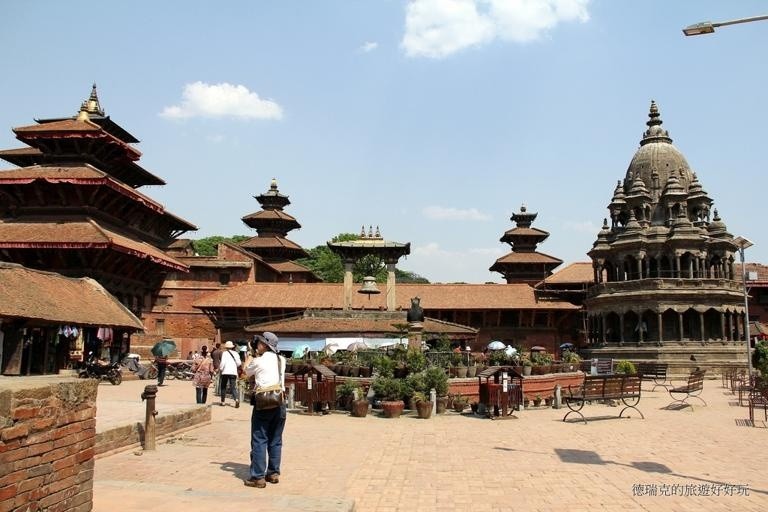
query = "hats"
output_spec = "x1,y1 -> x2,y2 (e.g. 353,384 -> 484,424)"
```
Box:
258,332 -> 278,353
224,341 -> 234,349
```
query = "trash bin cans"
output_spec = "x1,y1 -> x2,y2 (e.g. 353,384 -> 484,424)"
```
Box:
292,363 -> 337,413
478,365 -> 525,420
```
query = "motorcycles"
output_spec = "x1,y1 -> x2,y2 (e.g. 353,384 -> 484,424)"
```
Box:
78,350 -> 123,385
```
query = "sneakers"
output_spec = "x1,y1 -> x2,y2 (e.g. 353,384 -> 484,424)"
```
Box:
245,476 -> 266,488
235,400 -> 240,408
220,403 -> 226,405
266,473 -> 280,483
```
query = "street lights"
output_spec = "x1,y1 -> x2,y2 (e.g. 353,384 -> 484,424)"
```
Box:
700,233 -> 758,407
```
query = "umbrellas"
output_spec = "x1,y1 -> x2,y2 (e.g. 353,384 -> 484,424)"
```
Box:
150,340 -> 176,358
291,344 -> 310,359
347,342 -> 367,354
487,340 -> 506,351
322,343 -> 339,357
559,342 -> 574,347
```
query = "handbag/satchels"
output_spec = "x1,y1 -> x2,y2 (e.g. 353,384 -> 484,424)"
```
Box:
254,384 -> 284,409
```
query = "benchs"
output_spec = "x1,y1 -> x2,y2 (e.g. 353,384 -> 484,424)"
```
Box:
637,362 -> 669,392
666,370 -> 707,411
561,374 -> 644,424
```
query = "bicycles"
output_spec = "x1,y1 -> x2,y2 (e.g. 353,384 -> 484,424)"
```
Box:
142,356 -> 196,380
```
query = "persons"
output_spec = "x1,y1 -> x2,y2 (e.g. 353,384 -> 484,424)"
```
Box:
244,331 -> 287,488
453,344 -> 461,352
186,340 -> 248,409
634,321 -> 647,343
156,355 -> 168,385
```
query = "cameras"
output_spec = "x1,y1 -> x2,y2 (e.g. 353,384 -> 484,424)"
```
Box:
250,335 -> 258,347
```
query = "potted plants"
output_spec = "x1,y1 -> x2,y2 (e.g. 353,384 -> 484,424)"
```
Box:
284,323 -> 579,419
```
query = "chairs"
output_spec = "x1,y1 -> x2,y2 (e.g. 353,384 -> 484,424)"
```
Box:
721,365 -> 768,425
